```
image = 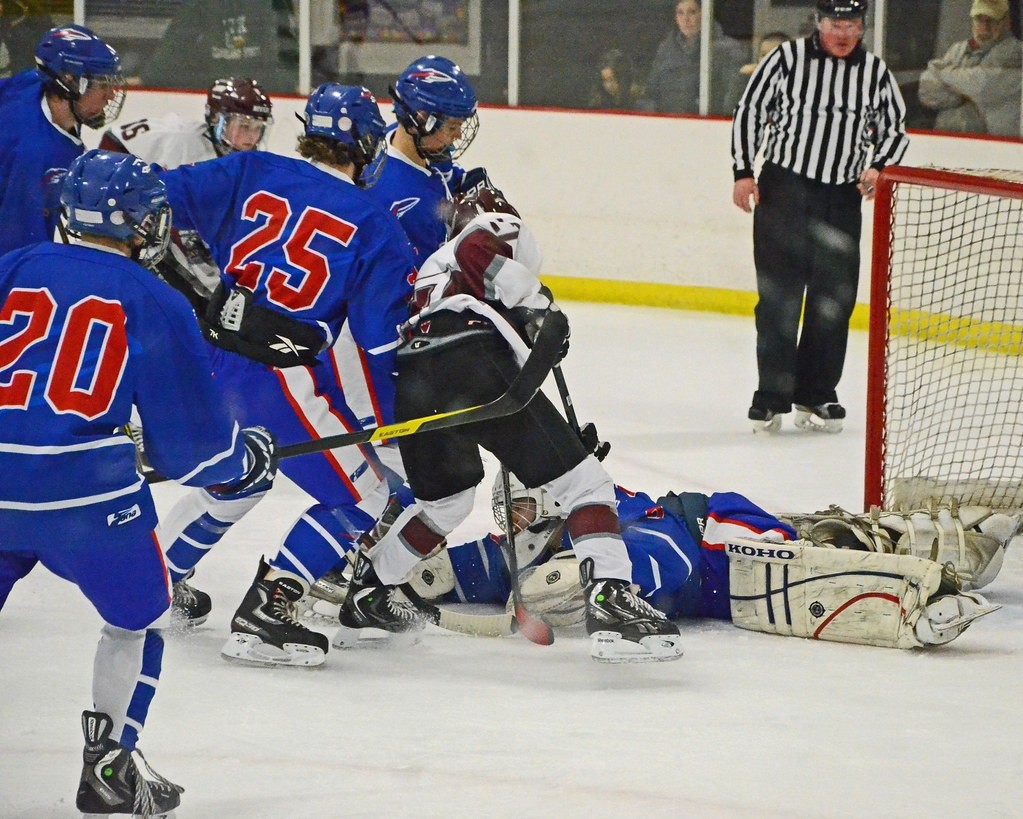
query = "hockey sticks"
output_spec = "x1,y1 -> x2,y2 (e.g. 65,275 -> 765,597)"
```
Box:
501,463 -> 556,645
143,311 -> 568,485
309,578 -> 430,613
553,362 -> 598,453
372,497 -> 519,636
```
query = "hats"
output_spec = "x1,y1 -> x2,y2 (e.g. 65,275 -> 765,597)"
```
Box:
969,0 -> 1010,21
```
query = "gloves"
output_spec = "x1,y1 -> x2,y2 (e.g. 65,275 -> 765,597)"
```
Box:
202,426 -> 278,495
524,284 -> 571,368
458,167 -> 508,203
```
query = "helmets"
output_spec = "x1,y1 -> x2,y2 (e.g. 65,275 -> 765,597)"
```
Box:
445,188 -> 521,242
815,0 -> 868,19
35,22 -> 129,130
304,82 -> 388,192
394,55 -> 480,164
57,149 -> 172,270
492,465 -> 561,524
204,76 -> 272,127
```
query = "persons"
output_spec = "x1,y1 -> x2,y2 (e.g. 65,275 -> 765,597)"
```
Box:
0,25 -> 1023,671
0,1 -> 293,96
730,0 -> 908,435
1,147 -> 277,819
590,0 -> 1023,139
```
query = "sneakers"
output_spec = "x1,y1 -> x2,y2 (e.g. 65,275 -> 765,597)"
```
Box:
167,567 -> 212,626
288,567 -> 353,628
747,406 -> 782,434
578,557 -> 686,664
914,591 -> 1003,645
968,513 -> 1021,554
76,710 -> 185,819
332,549 -> 427,650
220,554 -> 330,671
794,402 -> 846,434
